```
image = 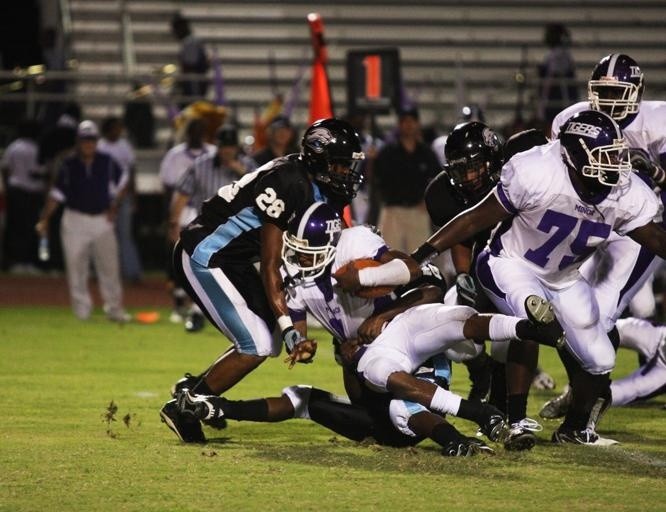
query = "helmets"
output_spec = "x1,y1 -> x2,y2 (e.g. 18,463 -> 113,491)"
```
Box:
302,118 -> 365,205
588,54 -> 645,122
280,200 -> 342,281
561,110 -> 633,188
444,121 -> 504,207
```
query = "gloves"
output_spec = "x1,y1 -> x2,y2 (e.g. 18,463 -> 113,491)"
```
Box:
630,147 -> 665,185
456,272 -> 479,308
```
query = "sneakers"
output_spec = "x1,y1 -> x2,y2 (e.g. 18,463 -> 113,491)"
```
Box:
159,372 -> 229,443
440,369 -> 619,456
524,295 -> 566,349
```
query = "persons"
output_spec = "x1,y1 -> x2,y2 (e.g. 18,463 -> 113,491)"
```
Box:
159,54 -> 666,455
539,20 -> 578,100
170,16 -> 211,106
2,104 -> 136,320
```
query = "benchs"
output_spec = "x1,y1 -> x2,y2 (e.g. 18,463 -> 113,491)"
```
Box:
58,1 -> 665,197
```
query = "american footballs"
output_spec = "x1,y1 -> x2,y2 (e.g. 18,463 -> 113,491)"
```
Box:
335,260 -> 394,300
